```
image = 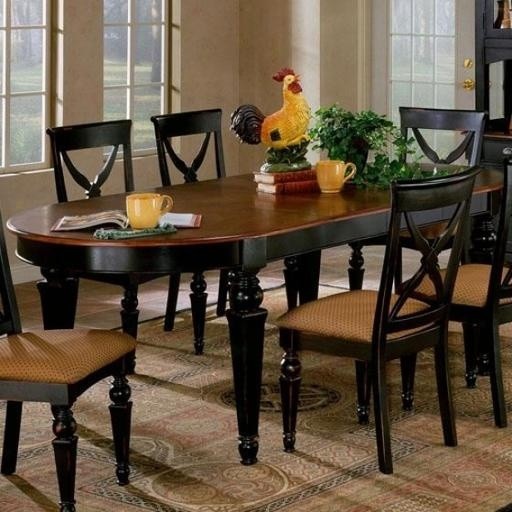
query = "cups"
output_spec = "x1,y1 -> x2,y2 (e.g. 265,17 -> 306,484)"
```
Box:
125,192 -> 174,232
315,160 -> 357,194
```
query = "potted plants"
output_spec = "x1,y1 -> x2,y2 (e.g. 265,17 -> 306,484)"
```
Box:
306,101 -> 467,190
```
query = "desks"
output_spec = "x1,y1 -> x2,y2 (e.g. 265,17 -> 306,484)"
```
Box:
2,163 -> 511,465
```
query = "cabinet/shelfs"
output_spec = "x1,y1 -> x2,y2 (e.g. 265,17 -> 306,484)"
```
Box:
475,0 -> 512,193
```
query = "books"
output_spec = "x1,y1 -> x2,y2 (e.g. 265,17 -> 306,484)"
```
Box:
50,208 -> 203,232
252,168 -> 318,184
253,180 -> 320,194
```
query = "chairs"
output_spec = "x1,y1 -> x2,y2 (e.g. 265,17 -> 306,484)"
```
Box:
401,160 -> 512,430
1,218 -> 140,511
274,160 -> 476,475
47,118 -> 206,359
148,108 -> 299,316
343,107 -> 490,293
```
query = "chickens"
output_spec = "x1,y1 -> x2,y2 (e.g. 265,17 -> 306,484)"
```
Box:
228,67 -> 313,166
